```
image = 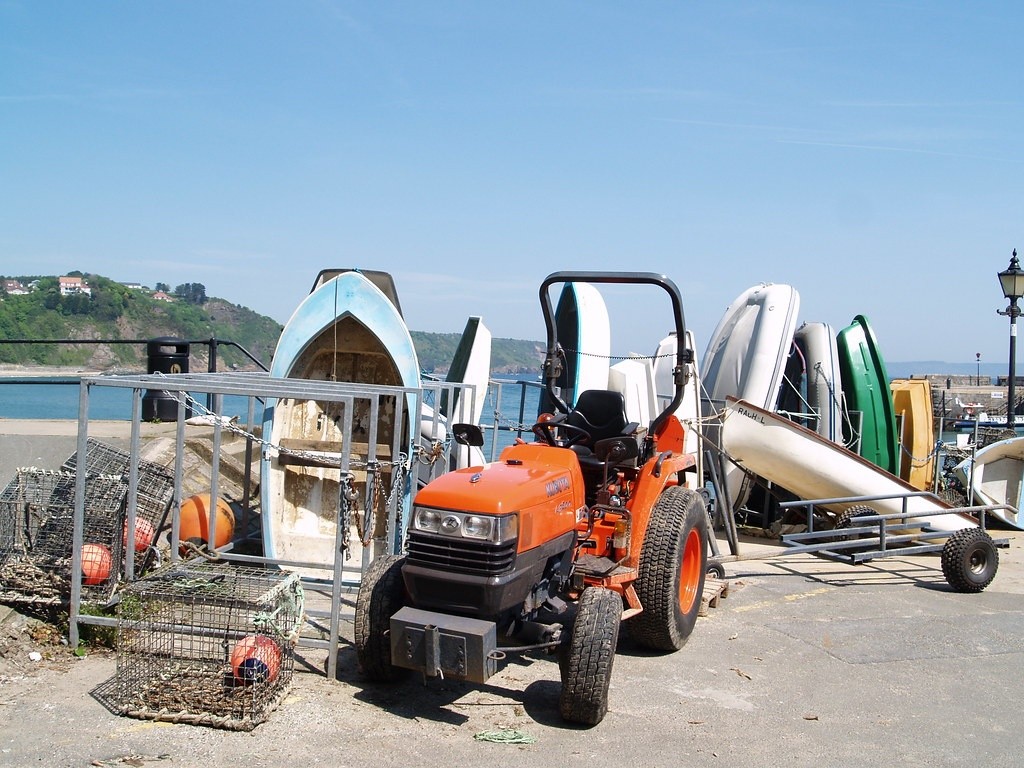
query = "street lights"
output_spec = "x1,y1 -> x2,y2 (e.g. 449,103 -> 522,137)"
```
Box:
995,246 -> 1024,433
975,352 -> 982,386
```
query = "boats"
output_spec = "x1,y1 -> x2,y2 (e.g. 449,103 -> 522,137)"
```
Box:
719,394 -> 982,546
889,377 -> 937,495
836,315 -> 901,479
607,352 -> 660,448
533,282 -> 612,443
652,328 -> 704,492
780,321 -> 846,448
951,437 -> 1024,533
257,264 -> 494,619
697,280 -> 800,530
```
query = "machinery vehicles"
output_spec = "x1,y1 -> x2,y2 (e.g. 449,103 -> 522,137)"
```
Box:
353,270 -> 711,729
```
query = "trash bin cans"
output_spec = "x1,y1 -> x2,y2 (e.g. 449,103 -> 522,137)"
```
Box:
141,336 -> 193,422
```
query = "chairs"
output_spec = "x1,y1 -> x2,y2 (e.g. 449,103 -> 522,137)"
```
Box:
552,390 -> 641,499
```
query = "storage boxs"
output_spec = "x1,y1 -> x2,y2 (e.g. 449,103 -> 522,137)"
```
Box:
1,435 -> 304,731
